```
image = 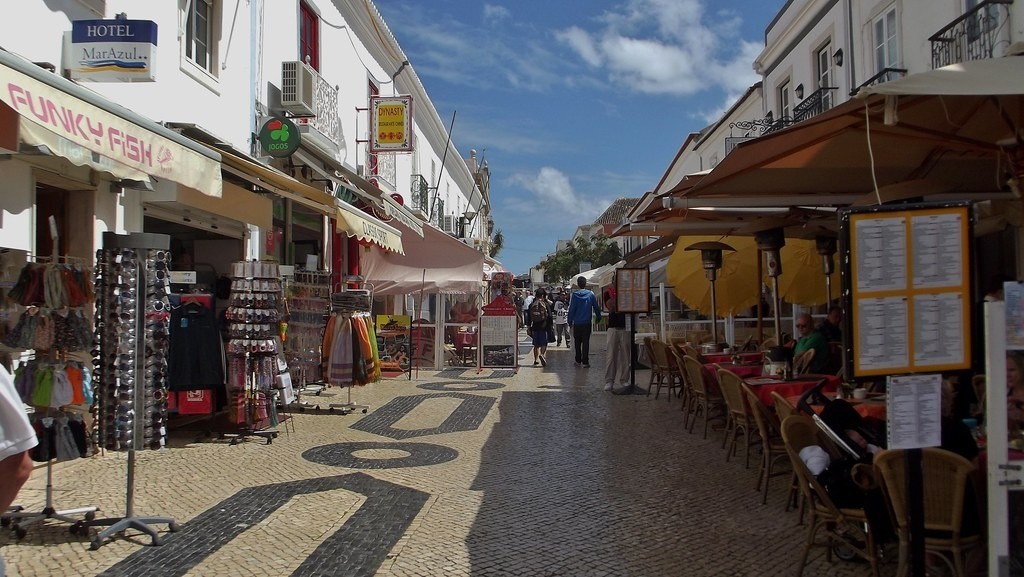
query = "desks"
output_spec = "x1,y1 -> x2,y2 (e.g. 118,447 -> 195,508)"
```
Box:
458,332 -> 476,350
702,352 -> 763,363
744,374 -> 841,406
703,361 -> 763,434
787,392 -> 886,427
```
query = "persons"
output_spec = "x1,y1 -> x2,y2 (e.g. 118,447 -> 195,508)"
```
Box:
511,285 -> 570,329
970,351 -> 1024,431
553,292 -> 571,348
772,306 -> 843,374
604,276 -> 630,391
846,430 -> 886,458
762,294 -> 770,317
977,275 -> 1003,372
567,276 -> 602,368
394,345 -> 406,362
0,363 -> 39,517
923,379 -> 988,577
527,288 -> 553,367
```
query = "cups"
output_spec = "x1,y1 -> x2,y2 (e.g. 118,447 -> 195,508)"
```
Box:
853,388 -> 865,399
723,348 -> 732,355
963,419 -> 977,441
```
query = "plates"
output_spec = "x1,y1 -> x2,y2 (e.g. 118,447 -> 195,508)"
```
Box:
871,395 -> 886,399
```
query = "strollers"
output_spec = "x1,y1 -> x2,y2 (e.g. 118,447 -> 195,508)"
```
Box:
795,378 -> 990,560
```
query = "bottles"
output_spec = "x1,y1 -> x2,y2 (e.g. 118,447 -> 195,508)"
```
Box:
784,360 -> 798,380
836,385 -> 844,399
687,341 -> 717,354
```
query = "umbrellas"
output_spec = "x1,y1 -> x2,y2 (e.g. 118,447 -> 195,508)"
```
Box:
761,238 -> 841,308
666,236 -> 765,318
609,42 -> 1024,238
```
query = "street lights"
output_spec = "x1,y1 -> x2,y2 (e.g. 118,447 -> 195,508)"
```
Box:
522,282 -> 525,288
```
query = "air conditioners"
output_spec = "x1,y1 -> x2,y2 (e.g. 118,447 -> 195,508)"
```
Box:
444,214 -> 456,235
281,61 -> 317,116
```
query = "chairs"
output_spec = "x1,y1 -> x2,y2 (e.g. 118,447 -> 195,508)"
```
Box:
643,334 -> 984,577
462,331 -> 477,366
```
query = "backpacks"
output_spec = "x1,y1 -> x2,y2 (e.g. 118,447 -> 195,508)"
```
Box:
530,298 -> 547,322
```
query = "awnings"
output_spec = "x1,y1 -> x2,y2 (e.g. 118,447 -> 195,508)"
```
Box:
0,45 -> 222,199
359,218 -> 501,371
293,136 -> 424,238
183,134 -> 405,256
565,255 -> 669,308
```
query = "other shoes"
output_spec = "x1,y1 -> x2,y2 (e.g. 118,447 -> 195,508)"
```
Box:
539,354 -> 546,367
582,364 -> 589,367
534,361 -> 539,366
575,361 -> 582,365
604,381 -> 613,390
619,382 -> 629,387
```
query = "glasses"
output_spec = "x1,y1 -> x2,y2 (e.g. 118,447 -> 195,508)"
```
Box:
89,247 -> 169,451
795,323 -> 807,328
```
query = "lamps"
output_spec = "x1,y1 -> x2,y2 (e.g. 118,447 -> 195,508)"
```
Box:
284,164 -> 333,191
456,212 -> 477,228
765,111 -> 772,123
795,83 -> 804,100
833,49 -> 843,67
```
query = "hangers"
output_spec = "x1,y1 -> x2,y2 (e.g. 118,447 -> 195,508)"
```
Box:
183,297 -> 202,313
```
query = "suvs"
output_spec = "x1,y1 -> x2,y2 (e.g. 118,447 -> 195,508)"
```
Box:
545,290 -> 566,314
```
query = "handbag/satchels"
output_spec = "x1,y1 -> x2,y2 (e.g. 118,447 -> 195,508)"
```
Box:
5,262 -> 94,462
